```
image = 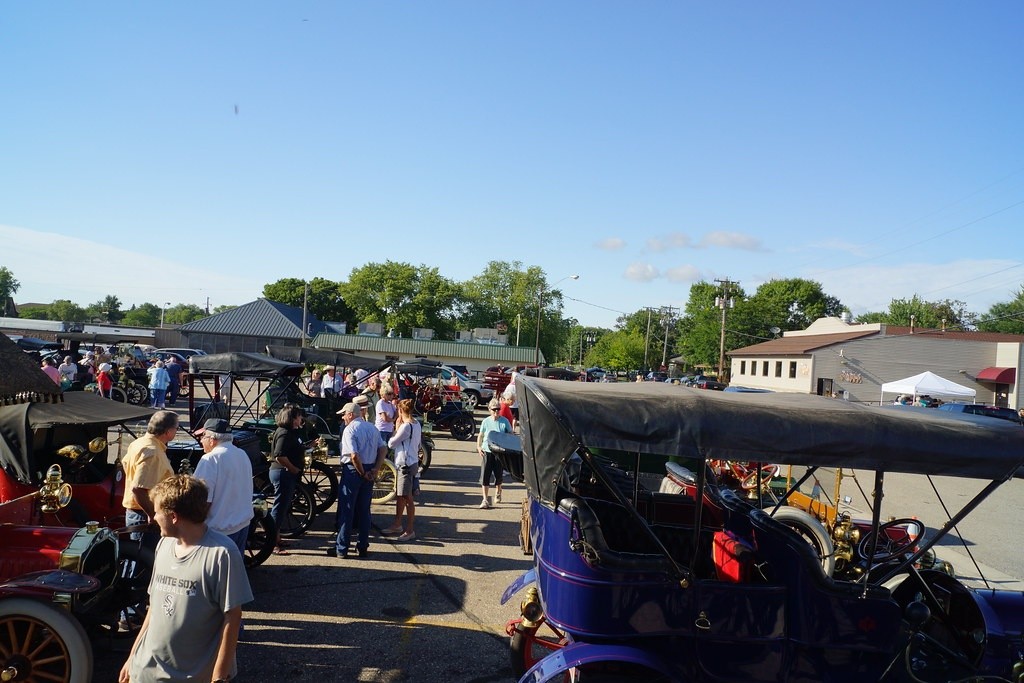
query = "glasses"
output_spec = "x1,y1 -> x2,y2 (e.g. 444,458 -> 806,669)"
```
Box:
172,424 -> 183,431
491,408 -> 500,412
386,392 -> 394,396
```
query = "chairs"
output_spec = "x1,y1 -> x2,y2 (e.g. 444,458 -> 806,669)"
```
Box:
721,486 -> 756,537
752,512 -> 895,633
668,464 -> 733,521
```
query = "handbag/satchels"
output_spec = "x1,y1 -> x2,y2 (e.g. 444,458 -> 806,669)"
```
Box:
396,463 -> 413,496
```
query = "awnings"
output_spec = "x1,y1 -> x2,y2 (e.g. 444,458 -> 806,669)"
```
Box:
976,367 -> 1015,383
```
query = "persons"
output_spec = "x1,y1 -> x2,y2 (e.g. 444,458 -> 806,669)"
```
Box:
602,375 -> 609,383
636,375 -> 642,381
450,371 -> 459,386
118,474 -> 254,683
477,371 -> 522,508
1019,409 -> 1024,417
895,394 -> 938,408
675,380 -> 697,387
194,418 -> 254,558
268,368 -> 422,558
148,357 -> 184,409
40,351 -> 112,398
119,411 -> 180,628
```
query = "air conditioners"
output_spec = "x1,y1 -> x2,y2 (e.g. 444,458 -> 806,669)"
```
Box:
454,331 -> 468,341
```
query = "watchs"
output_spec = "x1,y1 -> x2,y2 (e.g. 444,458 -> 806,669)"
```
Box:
212,679 -> 227,683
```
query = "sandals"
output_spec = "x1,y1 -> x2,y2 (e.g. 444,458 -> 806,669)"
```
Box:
275,540 -> 292,546
272,546 -> 290,556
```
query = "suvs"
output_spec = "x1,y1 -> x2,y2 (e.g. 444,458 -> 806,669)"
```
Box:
378,358 -> 495,410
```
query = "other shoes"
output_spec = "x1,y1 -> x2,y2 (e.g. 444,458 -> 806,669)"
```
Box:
480,500 -> 490,508
354,547 -> 367,557
381,525 -> 403,533
169,403 -> 175,406
495,489 -> 502,503
118,618 -> 142,630
398,530 -> 415,541
327,547 -> 348,559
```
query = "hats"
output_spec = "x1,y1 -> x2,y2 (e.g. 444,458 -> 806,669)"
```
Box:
336,402 -> 360,416
99,362 -> 112,372
352,395 -> 373,410
194,418 -> 232,435
322,365 -> 335,370
86,351 -> 95,356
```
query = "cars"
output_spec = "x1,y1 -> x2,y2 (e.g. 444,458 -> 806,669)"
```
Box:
937,402 -> 1024,425
77,342 -> 207,372
630,370 -> 774,394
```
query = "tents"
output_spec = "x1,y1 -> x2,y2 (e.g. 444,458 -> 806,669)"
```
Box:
880,371 -> 977,414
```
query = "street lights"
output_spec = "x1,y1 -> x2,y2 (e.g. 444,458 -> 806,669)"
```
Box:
534,275 -> 579,366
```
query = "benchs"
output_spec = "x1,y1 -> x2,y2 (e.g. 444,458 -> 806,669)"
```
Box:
272,387 -> 327,423
197,402 -> 256,445
586,459 -> 660,503
527,487 -> 690,639
